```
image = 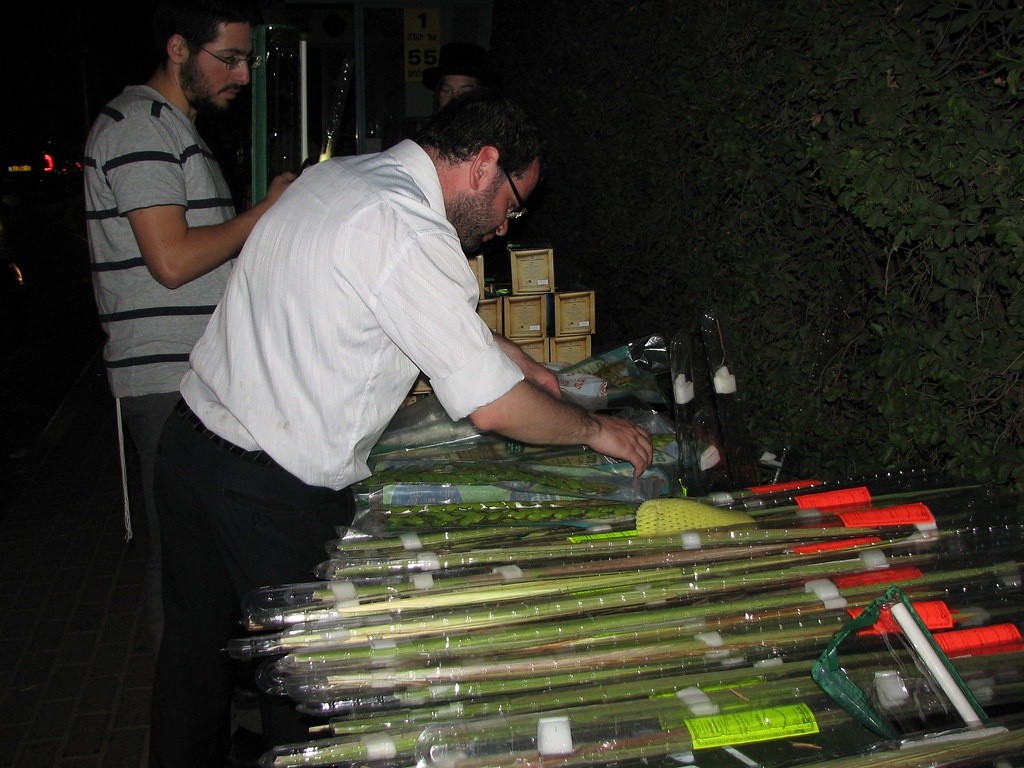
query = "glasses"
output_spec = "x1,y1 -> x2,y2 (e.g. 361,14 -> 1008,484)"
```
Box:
437,86 -> 465,98
185,38 -> 262,70
489,144 -> 527,219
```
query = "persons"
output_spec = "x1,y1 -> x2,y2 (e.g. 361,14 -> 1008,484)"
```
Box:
414,42 -> 530,276
85,0 -> 294,654
147,87 -> 653,768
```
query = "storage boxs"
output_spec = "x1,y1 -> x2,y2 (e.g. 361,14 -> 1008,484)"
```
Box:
548,289 -> 596,338
510,249 -> 554,295
477,296 -> 502,336
512,337 -> 549,364
411,374 -> 434,394
468,255 -> 486,300
549,334 -> 592,365
502,295 -> 547,338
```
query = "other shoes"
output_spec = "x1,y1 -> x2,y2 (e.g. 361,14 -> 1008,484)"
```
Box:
231,714 -> 272,763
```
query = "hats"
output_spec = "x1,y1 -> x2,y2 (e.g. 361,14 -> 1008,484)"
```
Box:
422,41 -> 503,93
